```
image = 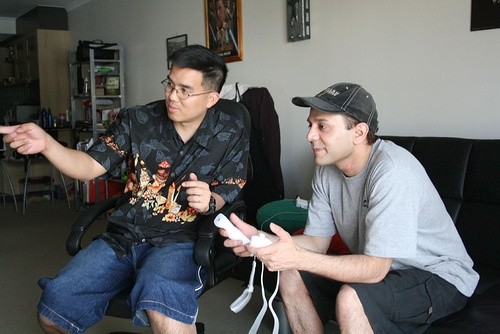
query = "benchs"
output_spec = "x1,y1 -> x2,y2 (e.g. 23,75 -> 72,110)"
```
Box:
372,135 -> 500,334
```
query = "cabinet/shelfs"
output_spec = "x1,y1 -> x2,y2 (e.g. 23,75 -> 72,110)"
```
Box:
4,30 -> 68,122
67,44 -> 126,201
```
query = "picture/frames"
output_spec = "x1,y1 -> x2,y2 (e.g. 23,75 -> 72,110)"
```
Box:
167,34 -> 186,69
203,1 -> 243,61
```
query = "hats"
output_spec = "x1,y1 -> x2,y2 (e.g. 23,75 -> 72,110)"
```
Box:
292,82 -> 379,132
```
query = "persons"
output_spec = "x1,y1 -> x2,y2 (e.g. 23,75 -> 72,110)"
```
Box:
0,46 -> 252,333
219,83 -> 480,334
208,0 -> 235,51
218,17 -> 227,50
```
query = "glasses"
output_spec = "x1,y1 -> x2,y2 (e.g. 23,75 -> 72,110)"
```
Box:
161,78 -> 215,99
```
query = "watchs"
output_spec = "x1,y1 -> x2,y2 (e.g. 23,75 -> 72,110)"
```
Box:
200,195 -> 216,216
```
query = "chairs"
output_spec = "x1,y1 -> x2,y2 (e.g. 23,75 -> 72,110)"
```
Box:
68,83 -> 275,319
